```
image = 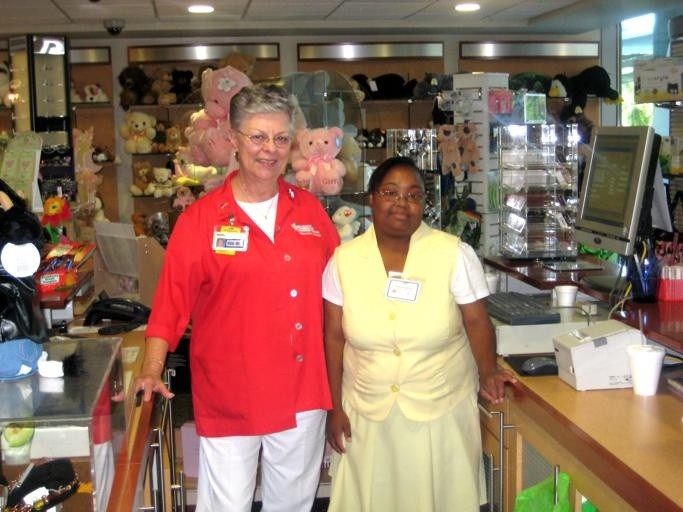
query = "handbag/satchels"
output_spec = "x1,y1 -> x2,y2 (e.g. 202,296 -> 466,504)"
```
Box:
0,278 -> 49,343
0,174 -> 53,279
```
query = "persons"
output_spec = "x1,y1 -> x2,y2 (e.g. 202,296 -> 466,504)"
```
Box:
322,158 -> 516,511
108,86 -> 343,512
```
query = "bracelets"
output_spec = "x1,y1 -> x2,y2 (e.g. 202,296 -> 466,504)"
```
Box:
143,357 -> 163,368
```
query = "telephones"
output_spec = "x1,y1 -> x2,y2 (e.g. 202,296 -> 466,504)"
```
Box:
83,298 -> 151,326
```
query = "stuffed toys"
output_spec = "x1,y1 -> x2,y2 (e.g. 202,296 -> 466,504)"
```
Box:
38,196 -> 72,244
70,51 -> 361,246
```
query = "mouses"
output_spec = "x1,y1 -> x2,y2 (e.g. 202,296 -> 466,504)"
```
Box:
521,355 -> 557,376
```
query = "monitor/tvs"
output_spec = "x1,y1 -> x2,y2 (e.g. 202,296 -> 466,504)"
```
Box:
573,125 -> 661,295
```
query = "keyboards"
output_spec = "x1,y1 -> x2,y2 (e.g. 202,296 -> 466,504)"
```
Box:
489,290 -> 561,326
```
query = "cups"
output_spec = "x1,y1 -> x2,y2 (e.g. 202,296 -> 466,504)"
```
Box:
555,286 -> 578,306
485,273 -> 499,294
629,343 -> 665,396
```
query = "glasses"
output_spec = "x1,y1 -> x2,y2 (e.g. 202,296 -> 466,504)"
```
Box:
237,129 -> 293,147
372,190 -> 427,204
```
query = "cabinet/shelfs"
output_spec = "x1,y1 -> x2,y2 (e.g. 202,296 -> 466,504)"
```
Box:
292,38 -> 601,266
0,291 -> 190,512
0,23 -> 116,285
123,38 -> 284,247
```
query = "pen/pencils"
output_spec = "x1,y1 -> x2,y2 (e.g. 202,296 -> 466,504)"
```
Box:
633,229 -> 657,300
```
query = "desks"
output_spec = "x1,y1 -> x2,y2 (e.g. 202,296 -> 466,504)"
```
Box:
475,353 -> 683,512
